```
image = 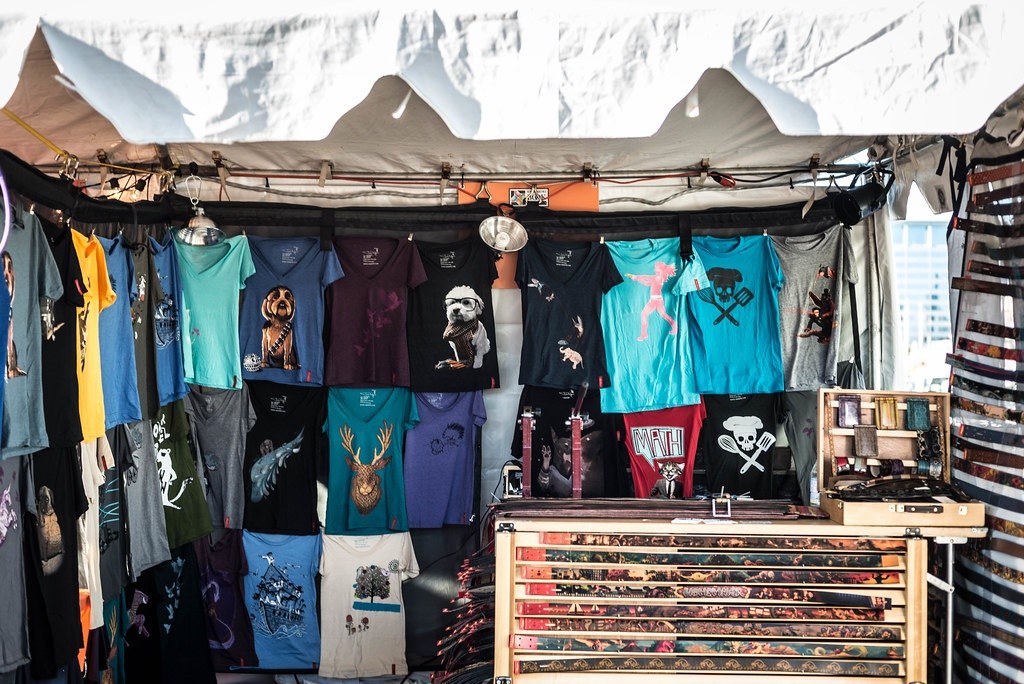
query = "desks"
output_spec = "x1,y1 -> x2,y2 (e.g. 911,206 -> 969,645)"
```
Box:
493,512 -> 988,684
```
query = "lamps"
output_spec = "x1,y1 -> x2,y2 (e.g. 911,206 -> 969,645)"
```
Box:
175,208 -> 227,246
479,215 -> 528,256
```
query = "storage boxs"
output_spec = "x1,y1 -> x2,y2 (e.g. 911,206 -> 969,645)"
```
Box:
816,387 -> 986,528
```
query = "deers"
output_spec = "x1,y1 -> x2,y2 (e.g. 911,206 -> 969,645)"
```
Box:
340,420 -> 393,515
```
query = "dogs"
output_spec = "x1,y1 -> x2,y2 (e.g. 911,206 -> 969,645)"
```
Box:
435,285 -> 490,371
259,286 -> 300,370
